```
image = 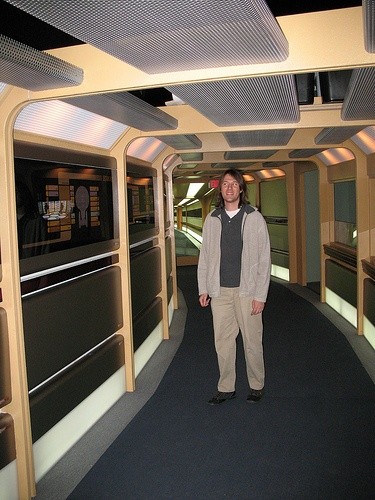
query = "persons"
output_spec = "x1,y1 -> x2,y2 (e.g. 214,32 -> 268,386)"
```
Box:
15,181 -> 49,295
197,169 -> 271,405
44,203 -> 49,214
60,203 -> 64,213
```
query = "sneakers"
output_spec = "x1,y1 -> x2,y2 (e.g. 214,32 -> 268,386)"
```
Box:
247,387 -> 265,403
208,391 -> 237,406
